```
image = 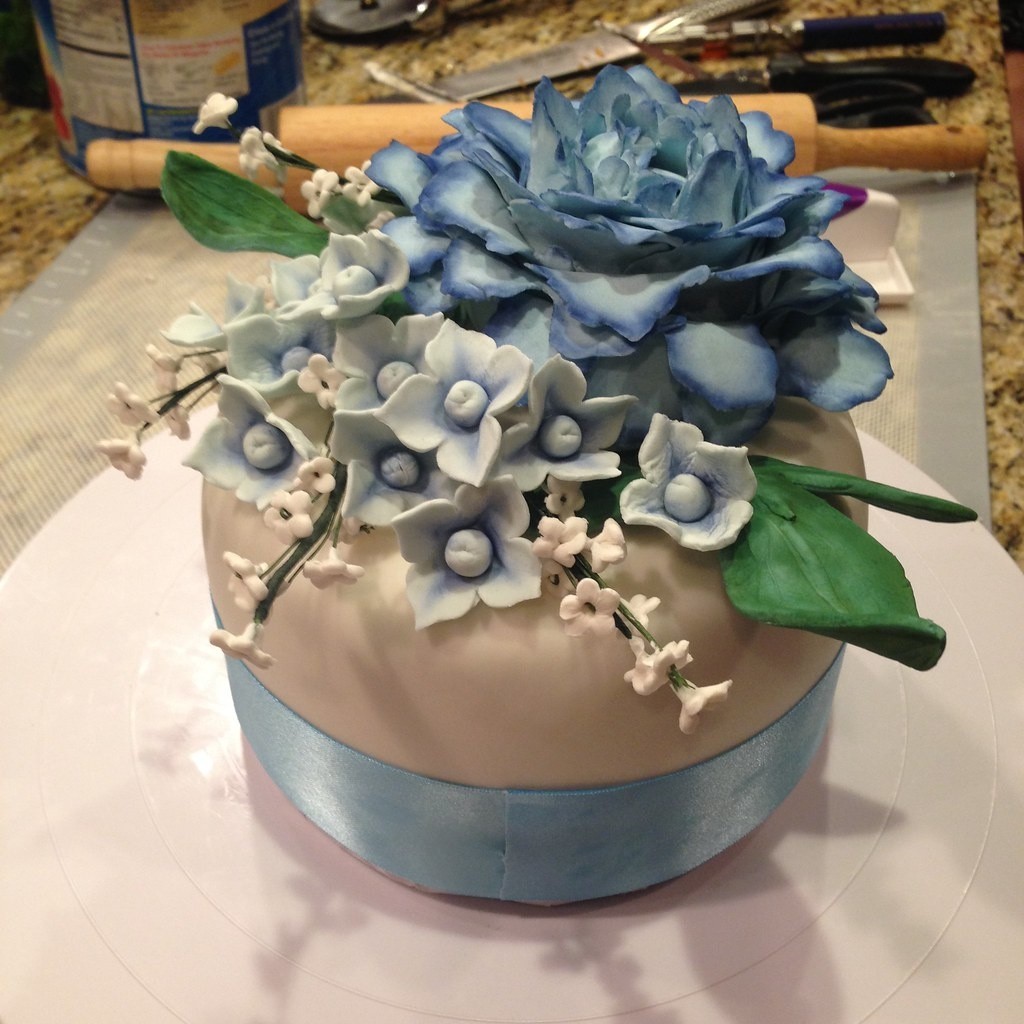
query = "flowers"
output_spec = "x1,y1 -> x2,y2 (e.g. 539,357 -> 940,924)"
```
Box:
92,50 -> 981,732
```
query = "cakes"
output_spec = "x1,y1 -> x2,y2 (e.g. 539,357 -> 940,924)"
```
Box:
97,64 -> 977,907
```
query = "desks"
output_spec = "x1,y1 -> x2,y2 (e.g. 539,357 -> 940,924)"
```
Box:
0,0 -> 1024,574
